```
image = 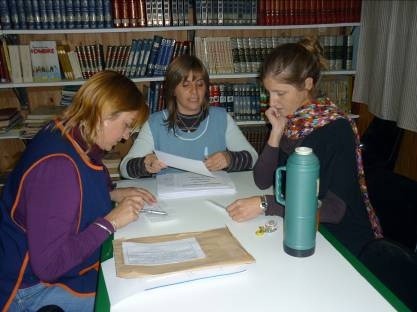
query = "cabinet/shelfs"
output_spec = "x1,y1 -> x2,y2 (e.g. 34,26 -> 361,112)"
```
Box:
0,22 -> 359,189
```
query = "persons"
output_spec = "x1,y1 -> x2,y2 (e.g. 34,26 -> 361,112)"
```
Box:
227,32 -> 384,259
0,71 -> 157,312
119,55 -> 258,180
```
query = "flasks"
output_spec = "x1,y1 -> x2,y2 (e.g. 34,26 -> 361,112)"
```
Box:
275,147 -> 319,257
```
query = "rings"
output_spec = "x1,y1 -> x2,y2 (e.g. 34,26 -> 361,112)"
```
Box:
150,161 -> 153,167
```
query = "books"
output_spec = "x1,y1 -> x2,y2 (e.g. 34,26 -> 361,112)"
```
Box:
0,0 -> 363,177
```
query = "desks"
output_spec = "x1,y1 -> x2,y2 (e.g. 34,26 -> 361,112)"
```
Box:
92,170 -> 415,311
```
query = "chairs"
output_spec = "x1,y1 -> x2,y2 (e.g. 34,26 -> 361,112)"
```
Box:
360,238 -> 417,312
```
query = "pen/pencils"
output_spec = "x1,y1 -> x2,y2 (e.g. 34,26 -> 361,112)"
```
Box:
205,199 -> 228,214
139,209 -> 168,215
204,146 -> 209,160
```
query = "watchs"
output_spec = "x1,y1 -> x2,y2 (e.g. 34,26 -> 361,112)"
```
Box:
259,195 -> 267,214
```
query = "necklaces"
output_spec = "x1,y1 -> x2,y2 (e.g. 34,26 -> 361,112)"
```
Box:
176,112 -> 202,133
